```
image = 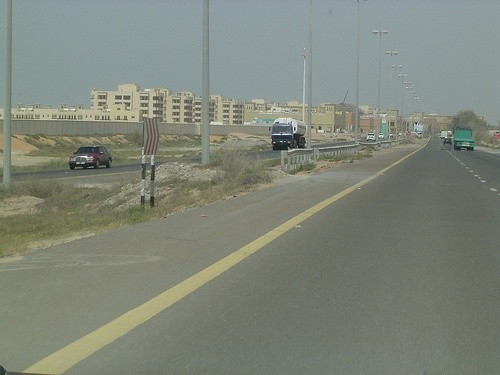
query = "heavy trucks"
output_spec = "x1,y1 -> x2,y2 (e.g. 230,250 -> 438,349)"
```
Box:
269,118 -> 306,151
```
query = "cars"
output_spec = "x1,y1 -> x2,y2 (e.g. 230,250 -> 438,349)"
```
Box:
367,133 -> 375,141
379,133 -> 383,139
69,146 -> 113,171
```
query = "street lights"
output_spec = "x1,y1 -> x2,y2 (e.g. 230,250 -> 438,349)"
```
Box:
385,50 -> 398,130
391,64 -> 422,132
372,28 -> 388,131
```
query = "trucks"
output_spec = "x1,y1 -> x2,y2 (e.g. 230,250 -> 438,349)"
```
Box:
454,129 -> 474,151
